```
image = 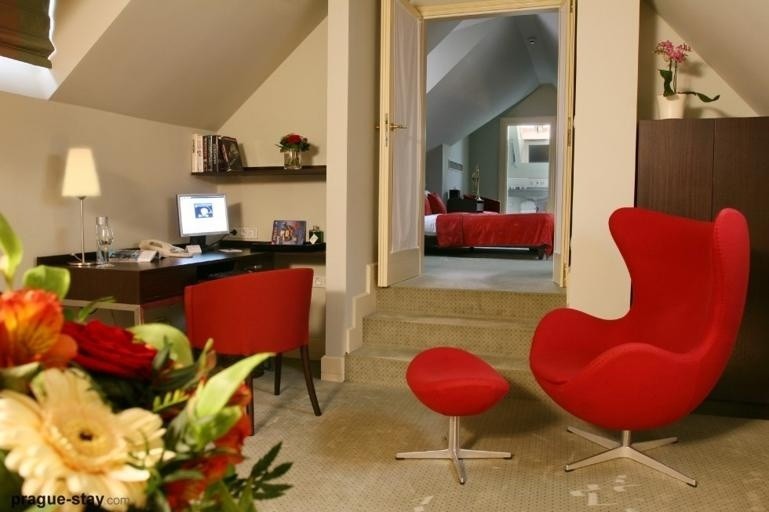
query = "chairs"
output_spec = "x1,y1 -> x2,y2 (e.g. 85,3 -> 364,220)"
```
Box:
529,205 -> 751,487
182,267 -> 325,439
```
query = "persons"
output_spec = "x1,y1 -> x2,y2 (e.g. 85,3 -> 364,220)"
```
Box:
279,223 -> 299,243
198,207 -> 210,218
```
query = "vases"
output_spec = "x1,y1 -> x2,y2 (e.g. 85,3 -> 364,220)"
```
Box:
654,93 -> 687,120
283,148 -> 304,170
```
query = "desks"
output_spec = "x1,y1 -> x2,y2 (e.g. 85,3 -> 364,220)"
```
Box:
35,246 -> 270,381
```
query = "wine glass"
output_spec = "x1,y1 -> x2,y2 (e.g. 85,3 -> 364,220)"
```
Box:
95,215 -> 113,270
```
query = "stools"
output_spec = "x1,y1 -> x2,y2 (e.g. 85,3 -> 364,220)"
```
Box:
395,345 -> 513,484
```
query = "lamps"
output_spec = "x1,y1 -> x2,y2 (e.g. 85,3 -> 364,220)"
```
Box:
58,145 -> 104,267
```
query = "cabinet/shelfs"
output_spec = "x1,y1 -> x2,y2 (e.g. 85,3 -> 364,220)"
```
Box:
189,165 -> 328,253
636,115 -> 769,420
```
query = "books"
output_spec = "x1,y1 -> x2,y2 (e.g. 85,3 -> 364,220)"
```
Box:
109,250 -> 164,263
192,133 -> 244,173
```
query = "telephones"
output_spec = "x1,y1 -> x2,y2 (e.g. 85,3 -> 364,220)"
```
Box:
138,240 -> 185,257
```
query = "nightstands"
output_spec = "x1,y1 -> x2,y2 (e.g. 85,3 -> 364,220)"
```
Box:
447,198 -> 484,213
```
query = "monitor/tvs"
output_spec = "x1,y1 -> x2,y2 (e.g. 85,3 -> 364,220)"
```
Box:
176,192 -> 229,247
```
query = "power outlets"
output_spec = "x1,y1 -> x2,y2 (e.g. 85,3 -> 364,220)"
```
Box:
228,228 -> 256,239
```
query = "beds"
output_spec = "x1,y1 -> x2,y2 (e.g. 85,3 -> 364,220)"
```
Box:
424,188 -> 554,260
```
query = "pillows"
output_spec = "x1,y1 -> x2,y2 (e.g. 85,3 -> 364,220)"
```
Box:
427,191 -> 448,214
424,197 -> 432,215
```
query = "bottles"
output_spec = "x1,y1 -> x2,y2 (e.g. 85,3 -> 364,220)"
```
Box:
309,225 -> 323,244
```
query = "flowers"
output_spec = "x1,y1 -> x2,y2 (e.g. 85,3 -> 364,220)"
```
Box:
0,212 -> 292,511
274,133 -> 311,152
649,38 -> 721,103
